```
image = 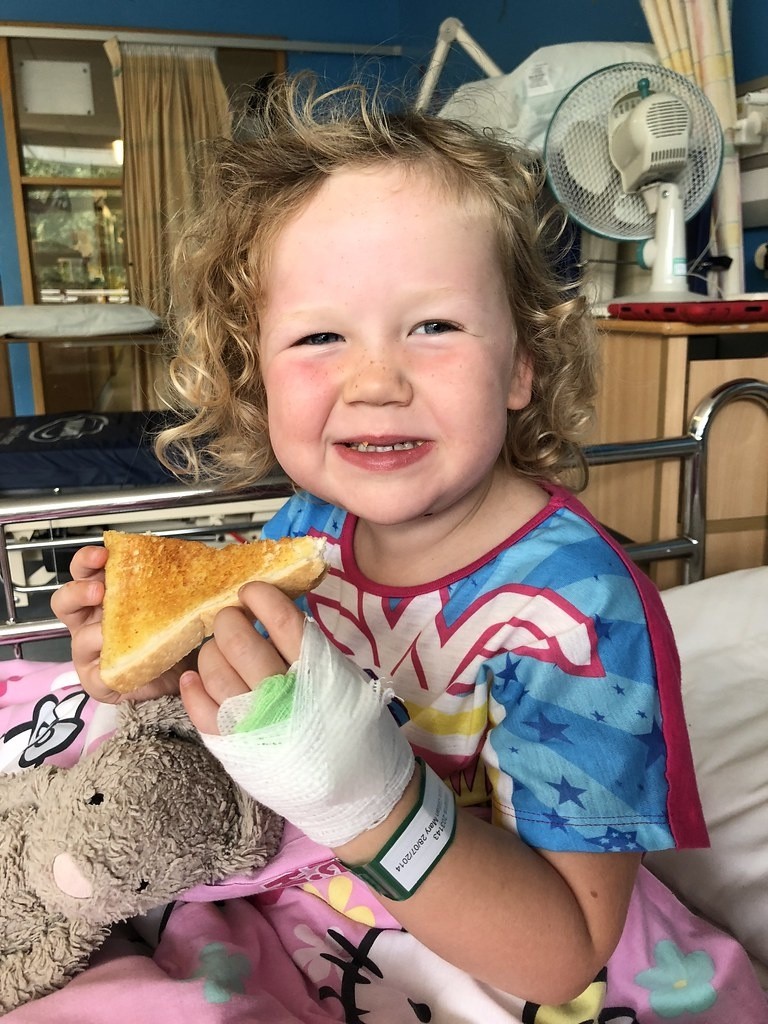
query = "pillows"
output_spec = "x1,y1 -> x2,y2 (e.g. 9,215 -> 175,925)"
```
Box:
0,303 -> 162,338
642,567 -> 768,968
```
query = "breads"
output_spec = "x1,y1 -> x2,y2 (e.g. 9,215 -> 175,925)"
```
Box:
98,528 -> 333,693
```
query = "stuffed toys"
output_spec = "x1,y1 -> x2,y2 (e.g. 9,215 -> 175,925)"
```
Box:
0,691 -> 282,1012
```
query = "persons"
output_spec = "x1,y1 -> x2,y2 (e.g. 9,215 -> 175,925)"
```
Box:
51,74 -> 713,1005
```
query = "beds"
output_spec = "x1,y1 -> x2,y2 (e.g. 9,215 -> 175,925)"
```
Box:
0,377 -> 768,1024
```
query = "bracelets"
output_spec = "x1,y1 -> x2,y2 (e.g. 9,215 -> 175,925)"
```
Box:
337,763 -> 456,898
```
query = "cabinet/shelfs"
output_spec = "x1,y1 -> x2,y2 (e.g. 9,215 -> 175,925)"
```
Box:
542,321 -> 768,588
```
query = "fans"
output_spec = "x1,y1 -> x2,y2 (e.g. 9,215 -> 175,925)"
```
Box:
542,62 -> 724,320
